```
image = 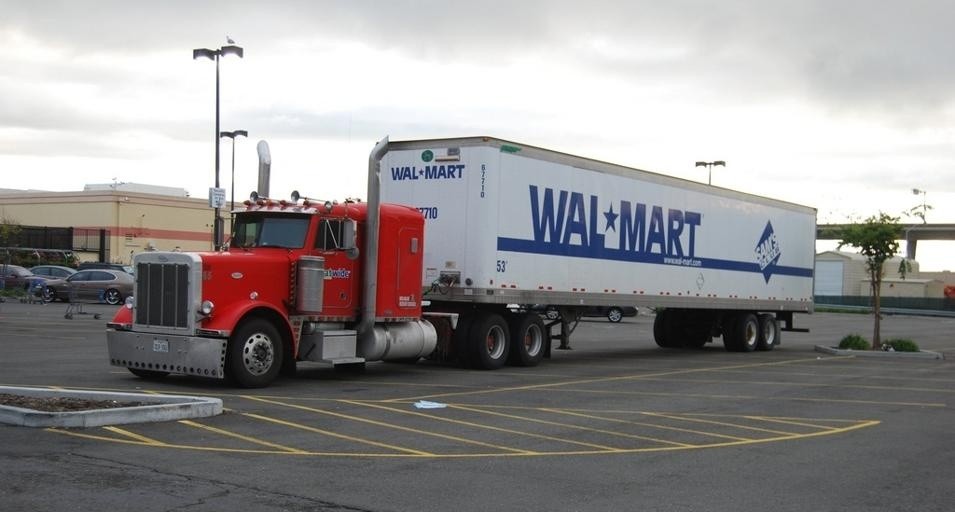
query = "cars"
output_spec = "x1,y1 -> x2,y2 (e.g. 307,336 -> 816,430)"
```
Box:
506,304 -> 640,323
0,262 -> 135,305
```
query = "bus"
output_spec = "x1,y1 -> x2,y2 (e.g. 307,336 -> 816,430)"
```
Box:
0,245 -> 82,270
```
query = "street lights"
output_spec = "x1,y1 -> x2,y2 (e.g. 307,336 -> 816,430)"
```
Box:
193,45 -> 247,252
694,160 -> 724,184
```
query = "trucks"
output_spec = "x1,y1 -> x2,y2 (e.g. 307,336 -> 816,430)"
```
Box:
106,136 -> 817,389
